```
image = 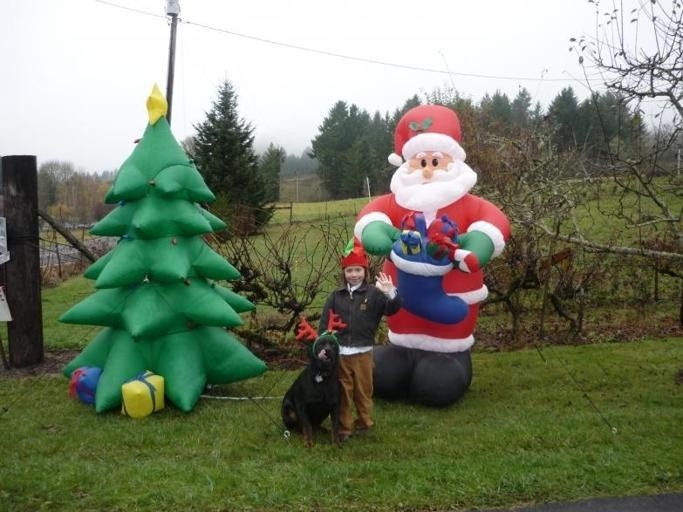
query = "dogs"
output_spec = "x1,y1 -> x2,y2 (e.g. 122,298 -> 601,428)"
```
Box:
280,334 -> 343,451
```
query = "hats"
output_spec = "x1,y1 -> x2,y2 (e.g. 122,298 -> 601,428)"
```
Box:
341,236 -> 368,269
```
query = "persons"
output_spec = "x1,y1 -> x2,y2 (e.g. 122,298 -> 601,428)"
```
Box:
319,235 -> 401,442
